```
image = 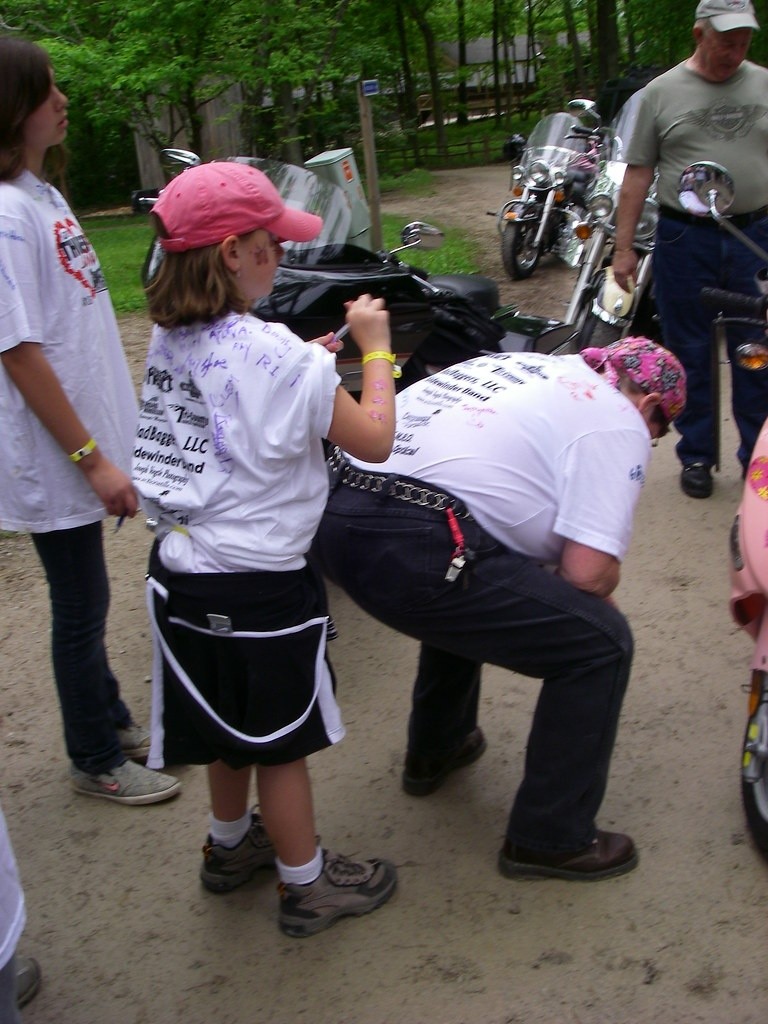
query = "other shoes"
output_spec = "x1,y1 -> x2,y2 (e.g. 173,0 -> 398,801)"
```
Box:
70,760 -> 182,805
12,956 -> 41,1005
117,720 -> 151,759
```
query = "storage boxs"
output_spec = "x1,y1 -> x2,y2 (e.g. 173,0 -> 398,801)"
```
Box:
304,148 -> 373,252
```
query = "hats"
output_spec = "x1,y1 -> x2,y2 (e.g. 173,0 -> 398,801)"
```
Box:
150,162 -> 322,252
695,0 -> 760,32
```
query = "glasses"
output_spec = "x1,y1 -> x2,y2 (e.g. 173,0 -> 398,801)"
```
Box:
648,433 -> 661,447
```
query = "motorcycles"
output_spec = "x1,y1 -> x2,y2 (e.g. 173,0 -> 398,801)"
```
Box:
563,125 -> 660,354
130,148 -> 579,403
676,160 -> 768,863
497,99 -> 602,283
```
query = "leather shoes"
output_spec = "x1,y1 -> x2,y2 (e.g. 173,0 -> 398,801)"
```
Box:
499,830 -> 638,880
402,727 -> 486,794
680,462 -> 713,498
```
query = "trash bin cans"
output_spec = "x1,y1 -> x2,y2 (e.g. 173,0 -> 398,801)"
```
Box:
304,146 -> 371,250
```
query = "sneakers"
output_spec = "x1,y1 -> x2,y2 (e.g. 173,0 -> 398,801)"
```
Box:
276,835 -> 397,938
200,803 -> 273,895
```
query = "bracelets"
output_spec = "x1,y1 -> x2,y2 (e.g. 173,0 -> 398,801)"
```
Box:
615,248 -> 634,252
68,439 -> 96,463
361,351 -> 401,379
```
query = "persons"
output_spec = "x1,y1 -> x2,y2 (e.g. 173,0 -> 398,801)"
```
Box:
319,337 -> 687,880
0,37 -> 180,804
130,161 -> 399,937
0,809 -> 41,1024
611,0 -> 768,497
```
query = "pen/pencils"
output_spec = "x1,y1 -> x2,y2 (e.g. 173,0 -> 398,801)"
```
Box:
324,319 -> 350,347
113,517 -> 125,534
